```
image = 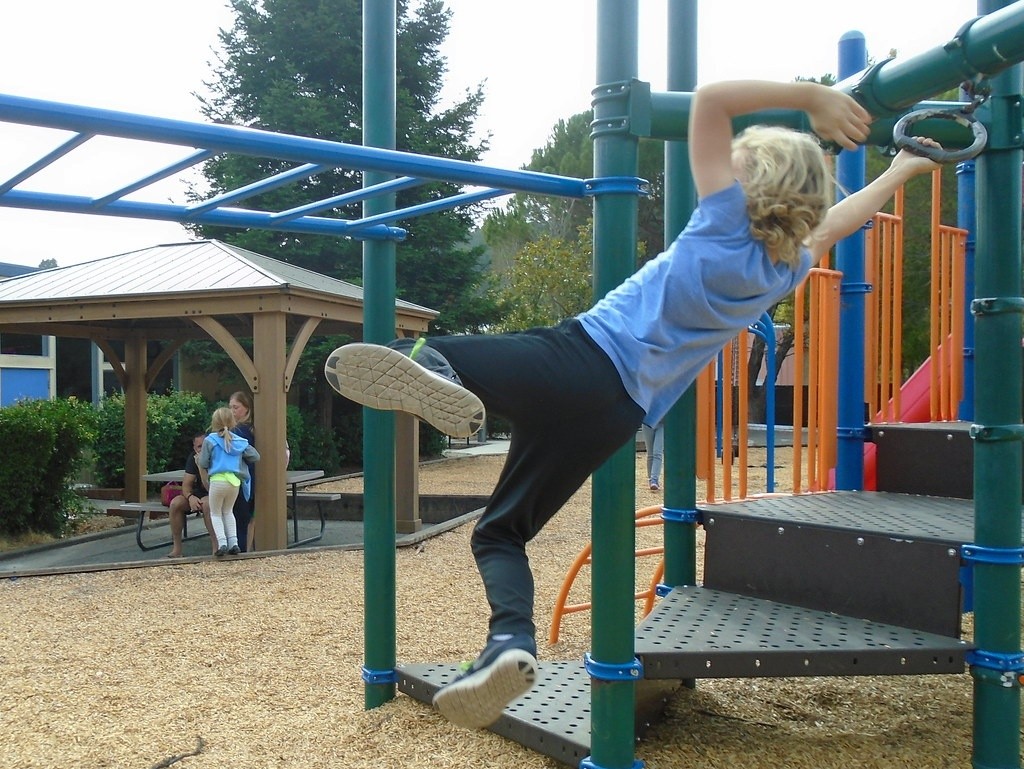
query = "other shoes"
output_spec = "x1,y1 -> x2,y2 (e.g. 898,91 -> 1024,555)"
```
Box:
323,338 -> 485,438
217,545 -> 226,556
431,633 -> 539,729
228,545 -> 241,554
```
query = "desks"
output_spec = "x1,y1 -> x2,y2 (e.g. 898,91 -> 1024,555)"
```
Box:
137,470 -> 326,551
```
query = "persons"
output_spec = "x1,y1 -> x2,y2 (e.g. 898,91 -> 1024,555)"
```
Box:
198,406 -> 260,557
227,389 -> 255,552
320,77 -> 944,733
642,420 -> 664,491
166,432 -> 220,557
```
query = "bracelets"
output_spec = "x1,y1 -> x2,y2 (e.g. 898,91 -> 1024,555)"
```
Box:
188,494 -> 194,501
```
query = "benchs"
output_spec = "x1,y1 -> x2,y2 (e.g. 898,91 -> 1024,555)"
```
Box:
121,502 -> 169,511
287,493 -> 341,502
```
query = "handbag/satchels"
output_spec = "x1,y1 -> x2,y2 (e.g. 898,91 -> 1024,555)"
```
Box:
161,481 -> 182,506
